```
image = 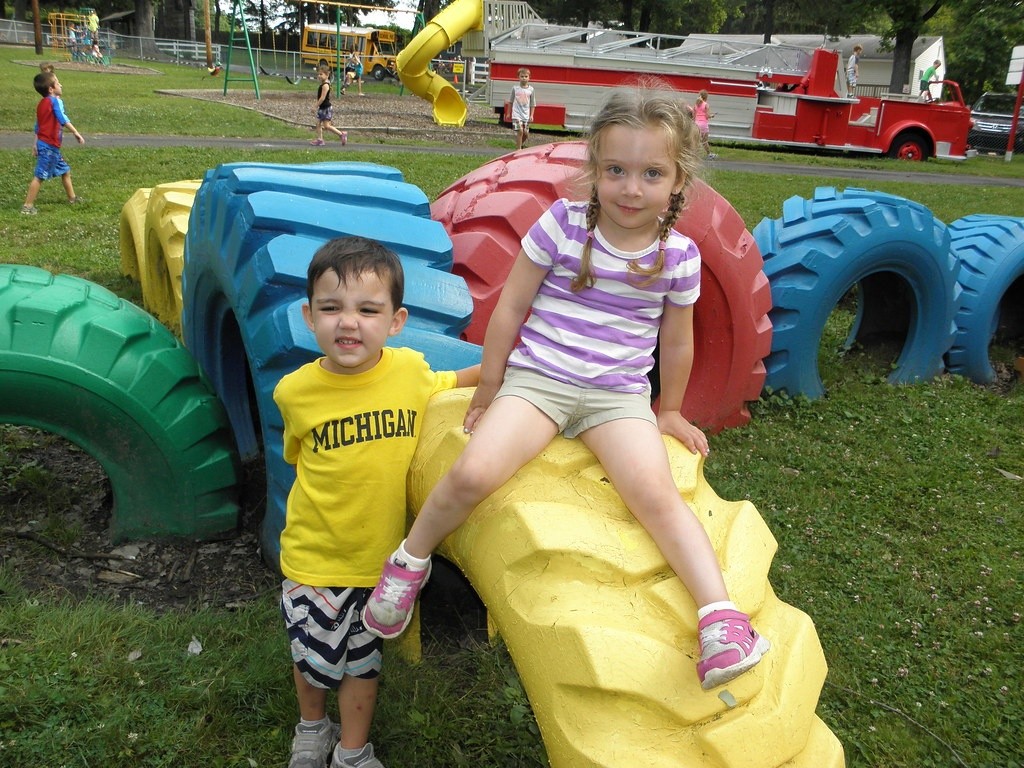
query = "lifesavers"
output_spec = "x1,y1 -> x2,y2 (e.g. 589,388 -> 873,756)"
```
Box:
0,134 -> 1024,768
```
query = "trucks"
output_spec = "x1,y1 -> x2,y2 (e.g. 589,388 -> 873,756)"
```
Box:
484,24 -> 974,162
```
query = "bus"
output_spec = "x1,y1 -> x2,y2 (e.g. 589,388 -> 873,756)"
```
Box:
301,24 -> 398,81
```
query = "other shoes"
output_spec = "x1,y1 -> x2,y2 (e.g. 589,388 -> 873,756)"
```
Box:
706,154 -> 717,159
340,90 -> 346,95
931,98 -> 936,102
358,93 -> 365,96
924,100 -> 932,102
847,94 -> 856,99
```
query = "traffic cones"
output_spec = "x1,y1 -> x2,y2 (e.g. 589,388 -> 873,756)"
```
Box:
454,75 -> 458,84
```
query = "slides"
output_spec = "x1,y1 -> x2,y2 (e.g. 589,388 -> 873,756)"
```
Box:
395,1 -> 485,127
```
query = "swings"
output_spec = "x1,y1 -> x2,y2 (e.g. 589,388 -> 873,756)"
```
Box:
257,0 -> 409,86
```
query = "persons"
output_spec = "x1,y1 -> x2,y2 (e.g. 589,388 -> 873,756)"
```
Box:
846,44 -> 863,99
919,60 -> 941,102
310,66 -> 348,146
20,73 -> 85,216
67,10 -> 104,57
360,83 -> 771,692
693,90 -> 718,159
273,236 -> 481,768
340,55 -> 363,95
773,82 -> 799,92
506,68 -> 536,150
758,74 -> 770,88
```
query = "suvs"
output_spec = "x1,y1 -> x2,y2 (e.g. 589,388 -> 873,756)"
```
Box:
968,93 -> 1024,154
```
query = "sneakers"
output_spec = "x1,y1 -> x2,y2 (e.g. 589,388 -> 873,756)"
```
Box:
330,740 -> 385,768
21,204 -> 37,215
288,714 -> 339,768
309,138 -> 325,146
362,556 -> 432,639
340,132 -> 347,146
69,195 -> 86,203
697,610 -> 772,690
